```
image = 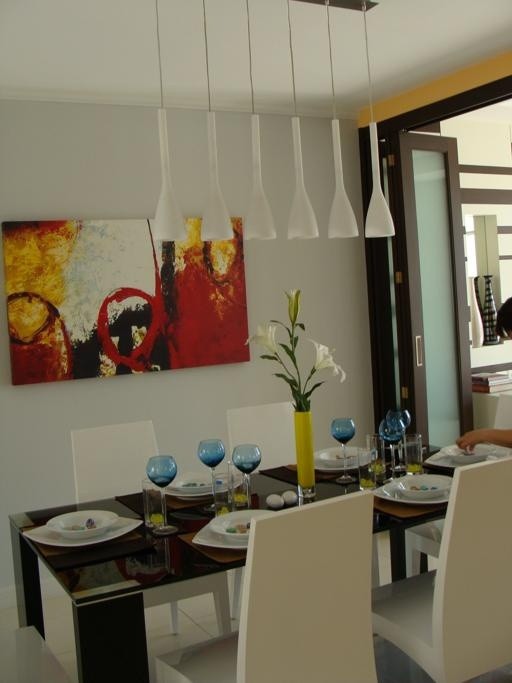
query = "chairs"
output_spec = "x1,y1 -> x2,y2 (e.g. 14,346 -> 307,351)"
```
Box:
404,393 -> 512,576
69,419 -> 232,639
0,625 -> 71,683
225,400 -> 296,476
154,490 -> 378,683
370,457 -> 512,683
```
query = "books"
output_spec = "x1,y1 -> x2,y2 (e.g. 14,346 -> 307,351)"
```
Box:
470,371 -> 512,393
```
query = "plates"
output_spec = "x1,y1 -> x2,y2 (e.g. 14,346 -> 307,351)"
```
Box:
191,509 -> 279,548
20,515 -> 142,548
424,440 -> 512,470
371,473 -> 454,505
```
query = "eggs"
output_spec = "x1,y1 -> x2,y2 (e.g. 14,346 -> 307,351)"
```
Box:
266,490 -> 298,509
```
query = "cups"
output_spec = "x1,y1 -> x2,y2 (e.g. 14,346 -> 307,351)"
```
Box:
144,533 -> 172,572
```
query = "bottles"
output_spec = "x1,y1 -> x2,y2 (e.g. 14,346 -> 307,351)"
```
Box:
466,273 -> 501,348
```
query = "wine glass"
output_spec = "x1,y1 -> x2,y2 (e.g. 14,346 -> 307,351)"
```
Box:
312,407 -> 426,494
140,437 -> 262,529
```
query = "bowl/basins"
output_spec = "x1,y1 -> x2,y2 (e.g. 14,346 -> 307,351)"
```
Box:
397,472 -> 452,499
46,508 -> 119,539
439,443 -> 495,464
208,511 -> 266,542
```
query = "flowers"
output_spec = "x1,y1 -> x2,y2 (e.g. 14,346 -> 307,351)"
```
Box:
245,288 -> 347,411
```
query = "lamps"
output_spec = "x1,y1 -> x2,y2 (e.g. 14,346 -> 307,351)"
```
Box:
150,0 -> 397,243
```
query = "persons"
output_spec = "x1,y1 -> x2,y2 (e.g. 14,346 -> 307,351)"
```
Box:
455,297 -> 512,452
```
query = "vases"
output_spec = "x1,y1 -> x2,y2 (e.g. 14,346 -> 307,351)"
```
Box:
466,274 -> 500,348
293,411 -> 317,498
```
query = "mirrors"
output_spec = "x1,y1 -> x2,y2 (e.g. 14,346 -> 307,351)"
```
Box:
461,215 -> 487,346
